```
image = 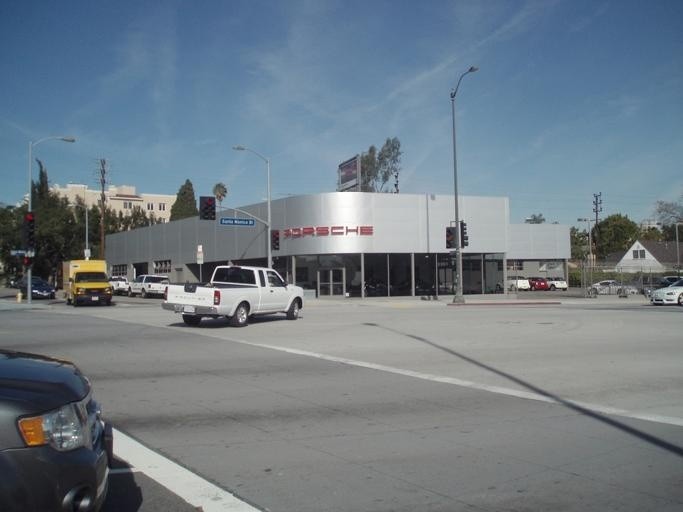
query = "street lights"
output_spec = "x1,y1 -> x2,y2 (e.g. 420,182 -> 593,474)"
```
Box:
575,217 -> 595,269
27,134 -> 74,301
451,66 -> 478,301
232,143 -> 272,273
67,202 -> 89,261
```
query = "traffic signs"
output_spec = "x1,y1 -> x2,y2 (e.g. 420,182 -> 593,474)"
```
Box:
220,217 -> 253,227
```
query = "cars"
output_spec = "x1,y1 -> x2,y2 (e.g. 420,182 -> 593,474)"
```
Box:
495,274 -> 567,292
4,274 -> 170,304
648,278 -> 682,307
362,278 -> 457,296
0,348 -> 113,510
586,275 -> 682,296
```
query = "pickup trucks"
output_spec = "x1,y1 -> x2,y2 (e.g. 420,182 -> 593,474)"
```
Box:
164,259 -> 305,324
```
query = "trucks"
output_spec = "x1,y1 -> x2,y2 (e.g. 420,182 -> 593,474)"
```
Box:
60,258 -> 114,307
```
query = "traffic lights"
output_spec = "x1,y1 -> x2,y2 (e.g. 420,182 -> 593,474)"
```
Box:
273,232 -> 278,251
445,228 -> 454,248
463,222 -> 467,246
22,256 -> 31,265
201,198 -> 215,219
26,213 -> 34,247
